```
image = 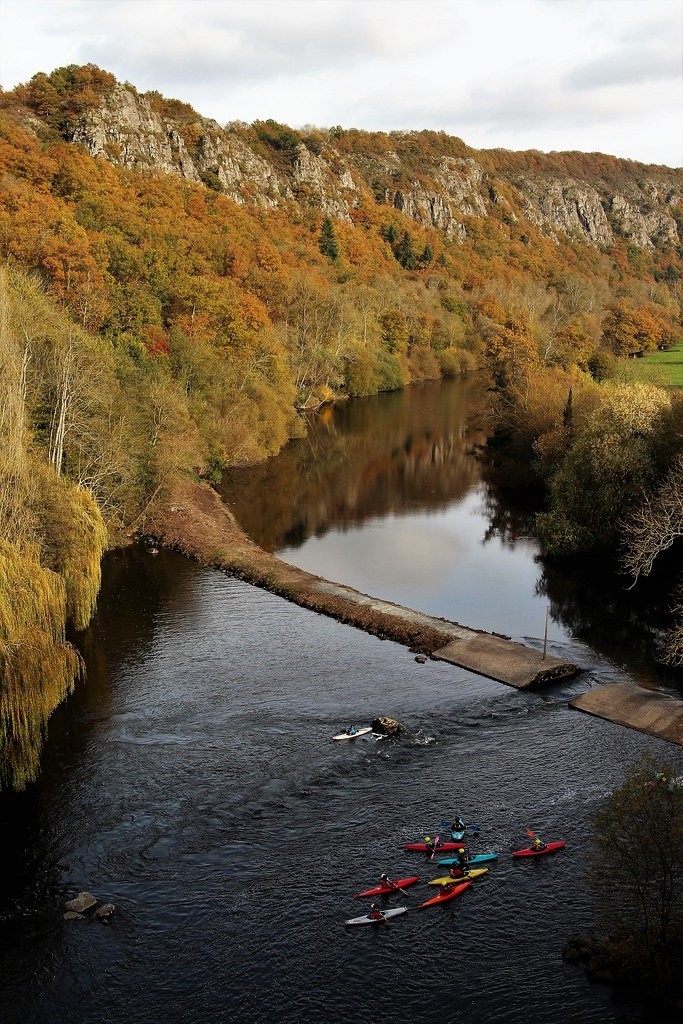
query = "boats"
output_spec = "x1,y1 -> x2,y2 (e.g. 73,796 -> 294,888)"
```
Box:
417,879 -> 474,908
344,906 -> 409,924
511,842 -> 565,857
451,816 -> 466,841
332,727 -> 373,740
428,868 -> 488,885
437,853 -> 499,865
354,875 -> 421,897
403,842 -> 467,851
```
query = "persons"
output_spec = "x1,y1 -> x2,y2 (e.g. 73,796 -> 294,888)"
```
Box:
347,725 -> 359,735
424,817 -> 473,899
533,839 -> 547,851
368,904 -> 383,920
380,874 -> 395,888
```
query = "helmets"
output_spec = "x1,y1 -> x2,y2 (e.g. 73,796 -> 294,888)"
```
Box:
452,862 -> 457,867
371,903 -> 379,909
459,848 -> 464,853
425,836 -> 430,842
534,839 -> 540,844
381,874 -> 387,880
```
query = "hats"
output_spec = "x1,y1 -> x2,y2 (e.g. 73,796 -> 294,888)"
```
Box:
455,817 -> 460,821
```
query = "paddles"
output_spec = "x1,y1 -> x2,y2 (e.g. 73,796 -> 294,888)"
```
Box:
379,913 -> 389,925
527,829 -> 551,849
387,878 -> 408,896
430,836 -> 439,859
